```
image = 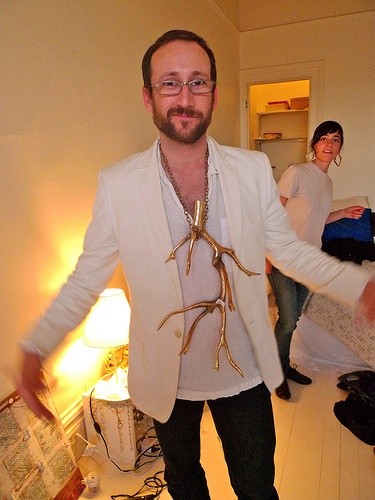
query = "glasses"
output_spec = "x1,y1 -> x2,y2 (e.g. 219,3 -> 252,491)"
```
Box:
149,77 -> 216,95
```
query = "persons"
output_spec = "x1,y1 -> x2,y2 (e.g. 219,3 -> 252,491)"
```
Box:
15,30 -> 375,500
265,121 -> 365,399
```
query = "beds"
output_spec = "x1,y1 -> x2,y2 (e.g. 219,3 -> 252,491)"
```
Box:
290,237 -> 375,374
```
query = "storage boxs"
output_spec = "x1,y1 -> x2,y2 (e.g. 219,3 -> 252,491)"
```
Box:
290,97 -> 309,110
265,101 -> 289,111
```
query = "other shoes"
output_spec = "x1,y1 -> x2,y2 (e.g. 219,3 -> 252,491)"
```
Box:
286,365 -> 312,385
275,381 -> 291,399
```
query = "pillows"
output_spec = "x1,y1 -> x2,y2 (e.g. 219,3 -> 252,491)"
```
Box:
324,208 -> 372,241
331,196 -> 370,212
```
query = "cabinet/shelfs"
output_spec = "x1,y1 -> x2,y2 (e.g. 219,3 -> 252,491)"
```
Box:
253,110 -> 308,151
82,385 -> 157,465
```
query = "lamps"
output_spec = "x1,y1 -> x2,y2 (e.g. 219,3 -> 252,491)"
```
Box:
84,289 -> 131,382
